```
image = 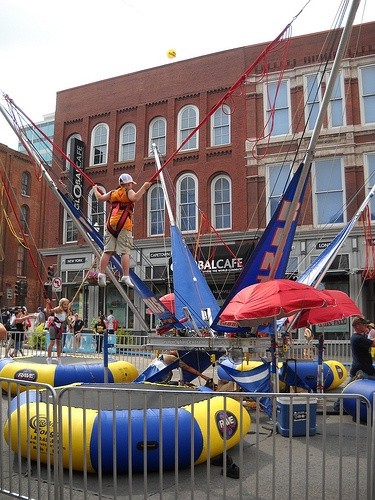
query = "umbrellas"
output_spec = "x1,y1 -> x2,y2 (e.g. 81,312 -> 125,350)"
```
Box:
148,293 -> 176,314
220,279 -> 338,429
284,290 -> 364,398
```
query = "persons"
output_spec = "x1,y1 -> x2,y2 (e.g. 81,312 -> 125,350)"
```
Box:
304,317 -> 375,410
92,174 -> 152,288
0,298 -> 115,365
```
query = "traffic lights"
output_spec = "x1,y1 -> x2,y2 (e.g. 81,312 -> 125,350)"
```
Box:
47,264 -> 55,282
14,280 -> 21,298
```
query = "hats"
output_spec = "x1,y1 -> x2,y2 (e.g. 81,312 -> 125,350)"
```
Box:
118,174 -> 137,185
352,317 -> 370,326
369,323 -> 374,327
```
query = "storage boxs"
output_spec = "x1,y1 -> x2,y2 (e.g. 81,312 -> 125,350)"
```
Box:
276,398 -> 317,437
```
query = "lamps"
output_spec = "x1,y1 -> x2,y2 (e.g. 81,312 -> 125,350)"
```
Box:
352,236 -> 358,252
300,239 -> 307,255
91,253 -> 96,267
137,249 -> 142,265
